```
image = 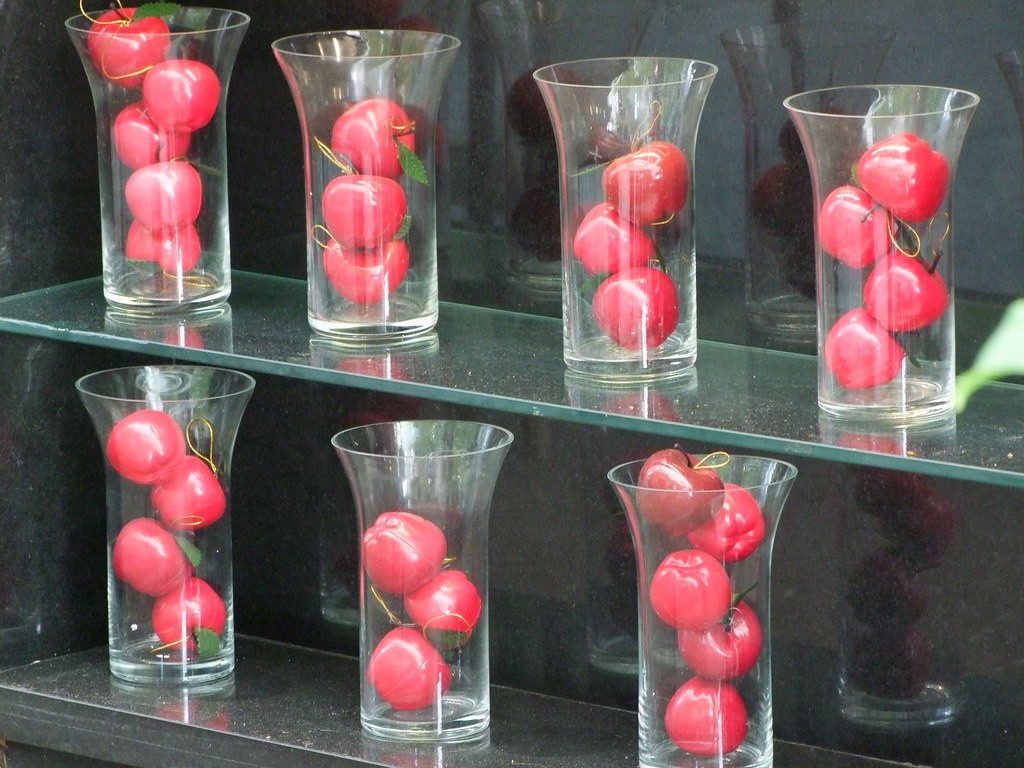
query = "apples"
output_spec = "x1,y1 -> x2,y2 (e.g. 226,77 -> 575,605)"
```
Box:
105,410 -> 224,649
323,98 -> 427,307
362,510 -> 481,713
820,131 -> 948,389
633,443 -> 765,754
89,6 -> 220,275
574,140 -> 686,348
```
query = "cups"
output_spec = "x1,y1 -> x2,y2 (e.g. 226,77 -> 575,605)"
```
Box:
719,20 -> 897,344
271,29 -> 461,342
478,0 -> 659,301
331,420 -> 514,741
783,84 -> 980,421
533,57 -> 719,376
586,424 -> 732,674
607,454 -> 799,768
65,7 -> 250,313
835,463 -> 976,728
317,380 -> 468,628
75,365 -> 256,683
